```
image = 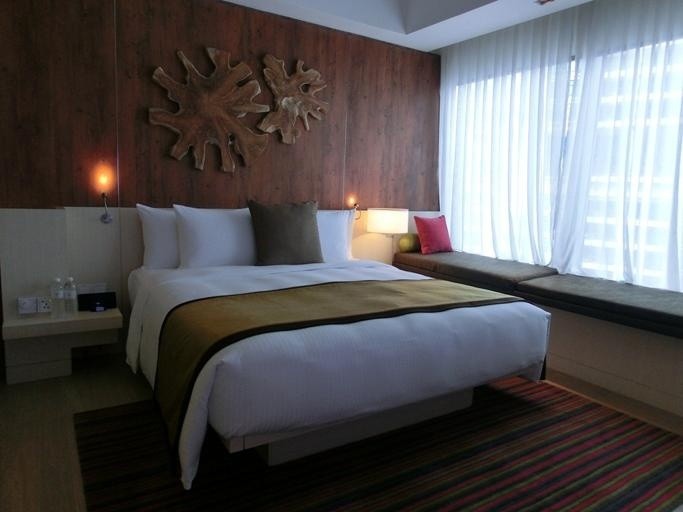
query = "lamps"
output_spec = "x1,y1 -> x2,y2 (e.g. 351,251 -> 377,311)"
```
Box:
88,161 -> 116,225
364,206 -> 410,241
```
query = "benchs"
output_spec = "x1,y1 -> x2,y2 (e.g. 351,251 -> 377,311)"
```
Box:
513,274 -> 682,425
396,251 -> 557,295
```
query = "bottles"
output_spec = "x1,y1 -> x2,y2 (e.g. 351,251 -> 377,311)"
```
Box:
50,277 -> 78,320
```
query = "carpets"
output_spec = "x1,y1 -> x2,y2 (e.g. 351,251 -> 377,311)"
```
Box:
72,380 -> 681,510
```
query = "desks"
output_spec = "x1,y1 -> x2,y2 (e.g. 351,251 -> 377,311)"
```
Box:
2,309 -> 121,345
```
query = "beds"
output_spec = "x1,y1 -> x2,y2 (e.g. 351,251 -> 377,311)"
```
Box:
127,257 -> 552,479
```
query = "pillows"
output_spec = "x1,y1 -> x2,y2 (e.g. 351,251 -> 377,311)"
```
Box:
135,200 -> 359,269
398,212 -> 451,257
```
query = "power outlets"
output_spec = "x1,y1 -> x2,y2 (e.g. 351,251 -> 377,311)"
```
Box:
38,297 -> 51,312
